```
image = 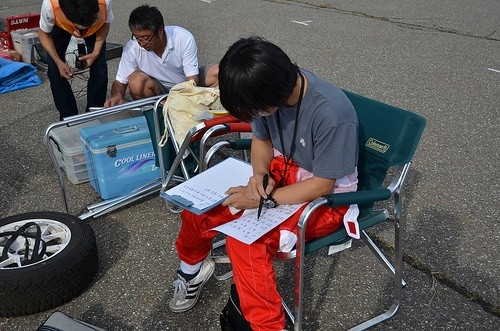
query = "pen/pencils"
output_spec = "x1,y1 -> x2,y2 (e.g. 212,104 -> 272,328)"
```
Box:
257,173 -> 269,219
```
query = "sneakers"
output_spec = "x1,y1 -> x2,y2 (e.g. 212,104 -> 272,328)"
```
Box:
170,262 -> 216,311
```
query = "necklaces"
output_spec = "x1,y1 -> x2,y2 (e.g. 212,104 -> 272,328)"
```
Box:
274,72 -> 305,187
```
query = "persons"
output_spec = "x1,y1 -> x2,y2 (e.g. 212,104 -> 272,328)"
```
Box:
104,4 -> 221,110
37,0 -> 116,122
170,35 -> 361,331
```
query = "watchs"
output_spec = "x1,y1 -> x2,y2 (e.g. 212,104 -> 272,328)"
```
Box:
262,193 -> 278,209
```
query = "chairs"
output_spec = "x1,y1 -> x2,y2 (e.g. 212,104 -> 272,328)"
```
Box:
44,94 -> 241,221
203,88 -> 427,331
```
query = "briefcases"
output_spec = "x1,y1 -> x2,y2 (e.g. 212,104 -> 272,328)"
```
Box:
77,114 -> 176,197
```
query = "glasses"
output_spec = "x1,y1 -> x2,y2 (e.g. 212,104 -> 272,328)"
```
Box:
131,31 -> 155,46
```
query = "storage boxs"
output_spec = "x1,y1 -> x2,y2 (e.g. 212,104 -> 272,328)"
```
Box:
10,27 -> 85,64
48,112 -> 133,184
79,117 -> 163,200
